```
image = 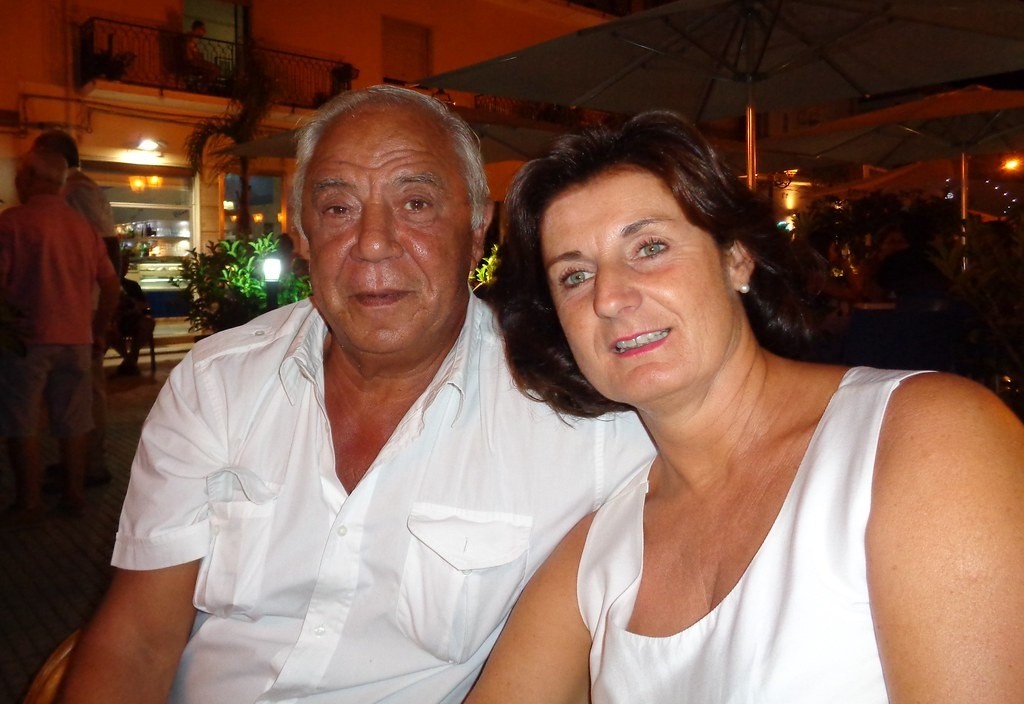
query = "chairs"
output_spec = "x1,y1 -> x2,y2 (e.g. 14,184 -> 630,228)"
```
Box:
147,307 -> 155,371
159,35 -> 209,94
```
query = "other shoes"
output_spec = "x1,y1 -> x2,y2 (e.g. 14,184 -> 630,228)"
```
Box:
116,360 -> 141,376
42,465 -> 71,492
0,503 -> 52,528
49,494 -> 88,517
78,469 -> 112,492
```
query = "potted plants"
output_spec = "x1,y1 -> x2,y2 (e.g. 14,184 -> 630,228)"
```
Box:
332,62 -> 360,99
94,50 -> 136,84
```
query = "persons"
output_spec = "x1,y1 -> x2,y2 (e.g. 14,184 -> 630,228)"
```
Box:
112,255 -> 150,371
50,86 -> 658,704
462,109 -> 1024,704
276,232 -> 307,277
792,199 -> 1024,377
186,20 -> 219,91
0,129 -> 122,522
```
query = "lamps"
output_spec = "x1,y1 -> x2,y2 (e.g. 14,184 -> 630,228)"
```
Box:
776,177 -> 812,211
